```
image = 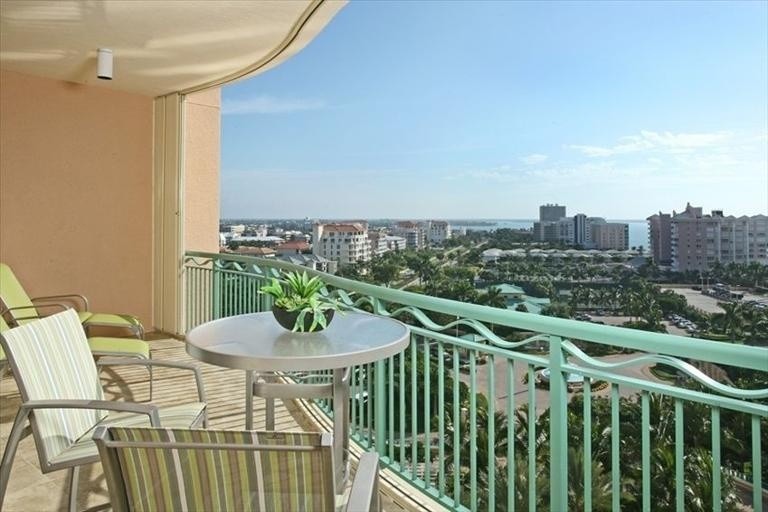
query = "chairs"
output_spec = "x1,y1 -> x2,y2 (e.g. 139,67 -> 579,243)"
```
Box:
0,263 -> 144,339
0,308 -> 205,510
0,315 -> 154,404
93,426 -> 383,512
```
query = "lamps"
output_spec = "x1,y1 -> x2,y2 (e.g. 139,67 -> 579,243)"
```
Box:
94,47 -> 115,82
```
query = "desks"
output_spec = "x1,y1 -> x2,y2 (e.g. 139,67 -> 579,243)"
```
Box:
181,311 -> 412,494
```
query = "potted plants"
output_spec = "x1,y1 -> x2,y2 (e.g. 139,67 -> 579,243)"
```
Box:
254,265 -> 351,333
